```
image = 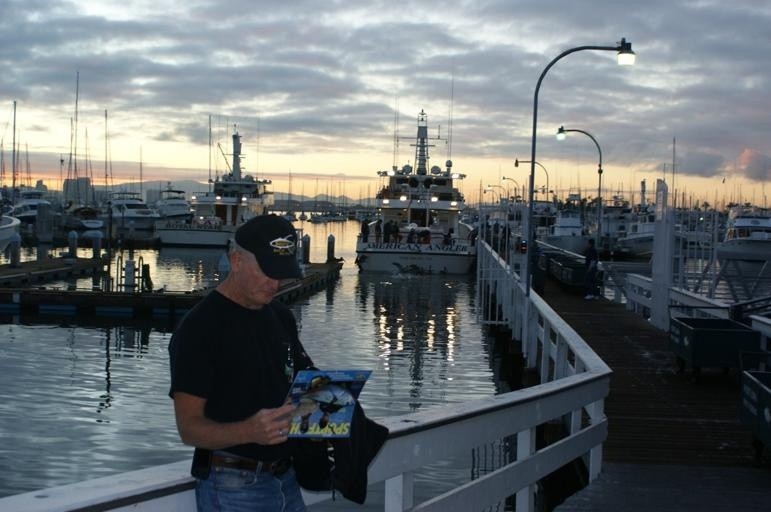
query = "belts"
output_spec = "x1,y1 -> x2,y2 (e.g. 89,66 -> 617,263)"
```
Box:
212,456 -> 291,477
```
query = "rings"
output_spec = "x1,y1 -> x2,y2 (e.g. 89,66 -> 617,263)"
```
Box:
277,427 -> 284,436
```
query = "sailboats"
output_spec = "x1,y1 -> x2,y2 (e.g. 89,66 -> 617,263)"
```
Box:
279,171 -> 346,223
0,68 -> 276,253
480,134 -> 771,268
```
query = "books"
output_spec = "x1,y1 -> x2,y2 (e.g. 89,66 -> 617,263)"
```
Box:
281,370 -> 372,438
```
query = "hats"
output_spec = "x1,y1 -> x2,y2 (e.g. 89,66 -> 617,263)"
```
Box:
235,214 -> 303,279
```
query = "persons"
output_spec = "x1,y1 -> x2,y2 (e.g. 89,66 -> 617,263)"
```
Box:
184,208 -> 244,232
166,212 -> 318,512
359,217 -> 600,301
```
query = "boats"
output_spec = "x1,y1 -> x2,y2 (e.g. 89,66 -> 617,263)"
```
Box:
355,106 -> 473,276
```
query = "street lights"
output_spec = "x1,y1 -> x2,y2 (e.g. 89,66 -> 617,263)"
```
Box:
524,37 -> 640,296
554,124 -> 606,250
